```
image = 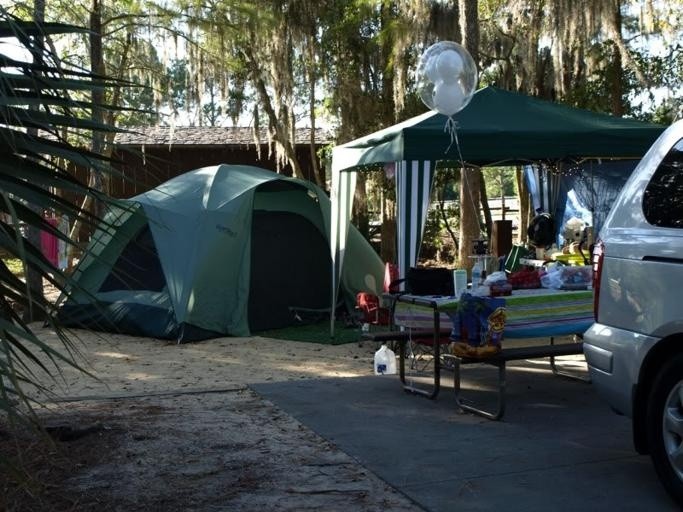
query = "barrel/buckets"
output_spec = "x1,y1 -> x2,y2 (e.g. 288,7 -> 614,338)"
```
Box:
373,345 -> 396,375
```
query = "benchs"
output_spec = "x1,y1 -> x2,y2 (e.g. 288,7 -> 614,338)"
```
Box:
448,341 -> 587,422
362,328 -> 463,385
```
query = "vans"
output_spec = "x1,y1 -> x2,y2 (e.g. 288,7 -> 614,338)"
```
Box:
580,115 -> 683,510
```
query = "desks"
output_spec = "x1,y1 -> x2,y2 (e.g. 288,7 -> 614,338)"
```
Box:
360,289 -> 598,400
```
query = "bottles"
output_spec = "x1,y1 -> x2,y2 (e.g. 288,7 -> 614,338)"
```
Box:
471,263 -> 482,293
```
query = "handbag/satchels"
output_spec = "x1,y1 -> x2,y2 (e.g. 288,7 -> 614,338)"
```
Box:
448,292 -> 505,356
505,212 -> 556,289
405,265 -> 452,295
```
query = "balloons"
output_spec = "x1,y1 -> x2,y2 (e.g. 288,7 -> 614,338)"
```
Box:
415,41 -> 477,116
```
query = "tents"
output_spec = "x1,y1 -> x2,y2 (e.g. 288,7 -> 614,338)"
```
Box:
41,163 -> 385,343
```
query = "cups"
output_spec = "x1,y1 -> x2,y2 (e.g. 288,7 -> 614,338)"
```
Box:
453,270 -> 468,299
